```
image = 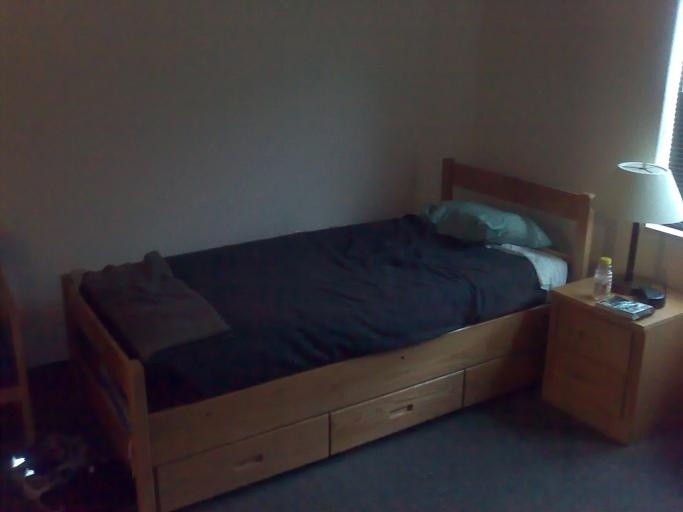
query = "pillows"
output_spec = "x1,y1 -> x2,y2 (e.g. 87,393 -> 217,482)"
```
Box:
81,251 -> 233,364
420,200 -> 553,250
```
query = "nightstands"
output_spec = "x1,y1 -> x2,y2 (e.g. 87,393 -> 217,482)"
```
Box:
542,273 -> 683,441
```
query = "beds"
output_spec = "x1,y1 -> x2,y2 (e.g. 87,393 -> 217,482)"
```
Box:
60,158 -> 597,512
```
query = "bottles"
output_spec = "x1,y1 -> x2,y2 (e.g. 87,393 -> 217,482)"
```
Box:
593,255 -> 615,302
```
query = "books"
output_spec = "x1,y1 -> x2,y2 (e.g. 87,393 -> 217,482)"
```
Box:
596,295 -> 655,322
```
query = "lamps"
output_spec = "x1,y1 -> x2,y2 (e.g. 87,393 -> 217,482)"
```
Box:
597,161 -> 683,294
